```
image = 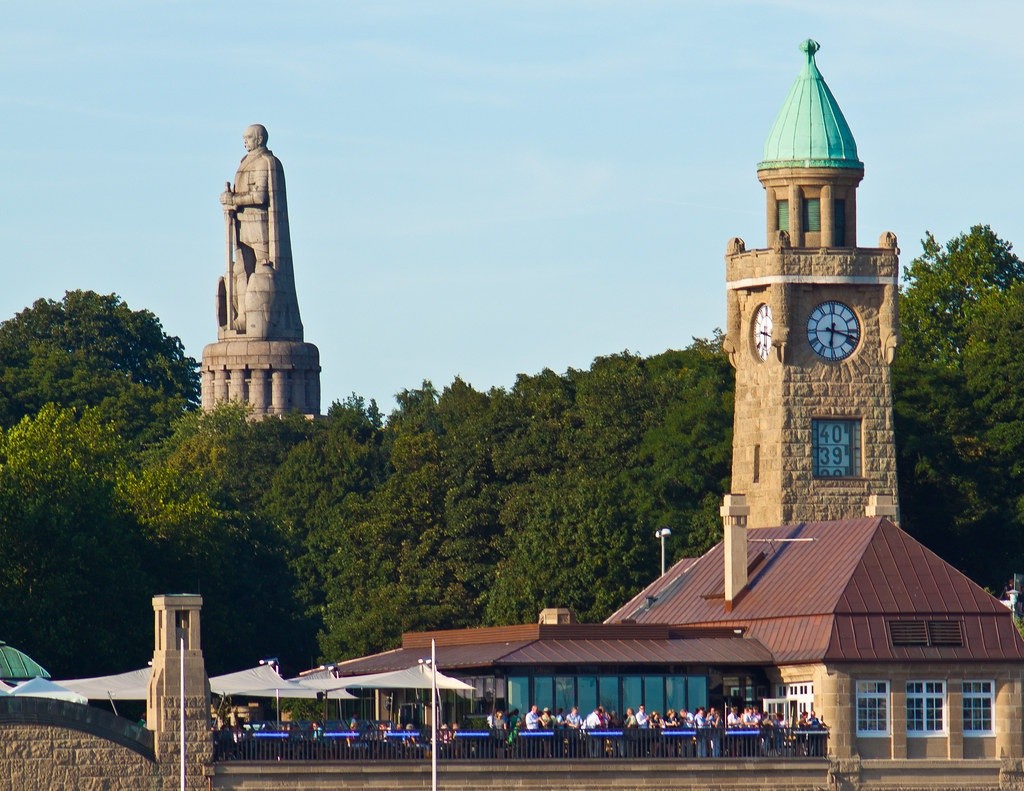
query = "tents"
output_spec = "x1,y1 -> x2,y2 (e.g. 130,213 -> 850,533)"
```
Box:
0,661 -> 479,724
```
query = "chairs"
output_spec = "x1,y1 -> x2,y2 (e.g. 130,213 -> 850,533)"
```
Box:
238,720 -> 433,759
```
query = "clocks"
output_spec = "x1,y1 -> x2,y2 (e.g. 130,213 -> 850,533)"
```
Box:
750,304 -> 773,364
806,300 -> 861,361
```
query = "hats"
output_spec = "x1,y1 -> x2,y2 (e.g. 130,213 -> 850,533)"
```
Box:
542,707 -> 548,712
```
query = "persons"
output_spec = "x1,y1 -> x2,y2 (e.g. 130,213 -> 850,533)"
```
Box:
214,718 -> 460,759
219,124 -> 303,333
486,704 -> 829,758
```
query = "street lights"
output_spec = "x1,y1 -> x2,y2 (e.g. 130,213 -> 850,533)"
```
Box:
321,665 -> 335,731
259,657 -> 279,728
655,528 -> 671,576
1007,590 -> 1019,623
418,638 -> 437,791
